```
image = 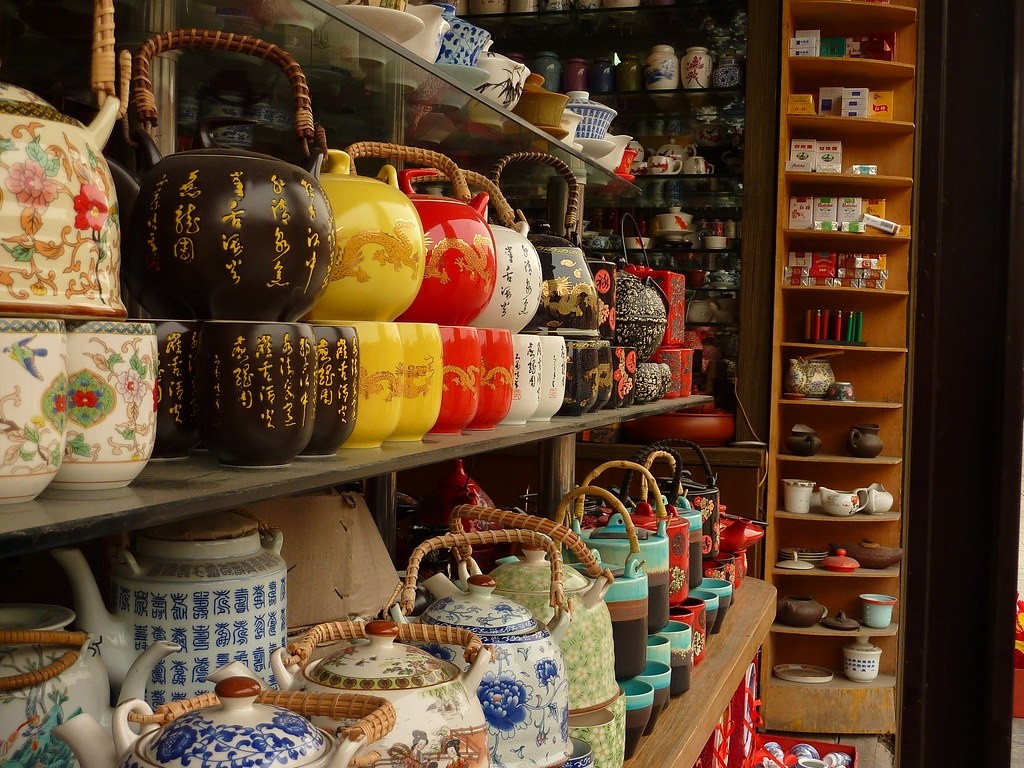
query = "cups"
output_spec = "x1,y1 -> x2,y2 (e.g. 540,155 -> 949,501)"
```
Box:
540,206 -> 740,322
760,742 -> 852,768
562,548 -> 747,768
841,637 -> 882,683
0,322 -> 693,511
781,479 -> 816,514
181,0 -> 639,185
824,383 -> 856,403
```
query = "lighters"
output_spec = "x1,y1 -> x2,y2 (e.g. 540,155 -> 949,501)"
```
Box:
801,308 -> 864,343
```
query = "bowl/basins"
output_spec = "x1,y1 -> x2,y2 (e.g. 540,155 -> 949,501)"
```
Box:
859,594 -> 897,629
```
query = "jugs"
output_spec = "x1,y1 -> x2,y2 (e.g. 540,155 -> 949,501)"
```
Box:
849,424 -> 883,458
788,424 -> 822,456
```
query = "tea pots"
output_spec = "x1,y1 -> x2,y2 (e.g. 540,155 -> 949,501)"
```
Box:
782,595 -> 824,627
630,138 -> 714,176
0,0 -> 666,361
859,483 -> 893,515
819,487 -> 869,517
786,350 -> 845,400
1,467 -> 763,768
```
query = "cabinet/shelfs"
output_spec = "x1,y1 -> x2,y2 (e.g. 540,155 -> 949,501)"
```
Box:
0,1 -> 922,768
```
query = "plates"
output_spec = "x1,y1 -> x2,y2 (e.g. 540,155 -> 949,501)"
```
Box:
779,548 -> 829,560
